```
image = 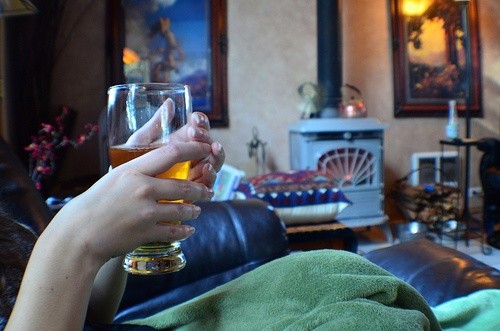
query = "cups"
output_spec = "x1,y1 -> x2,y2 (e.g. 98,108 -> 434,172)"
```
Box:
107,82 -> 194,274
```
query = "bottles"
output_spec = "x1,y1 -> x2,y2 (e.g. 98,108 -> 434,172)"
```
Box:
447,100 -> 460,138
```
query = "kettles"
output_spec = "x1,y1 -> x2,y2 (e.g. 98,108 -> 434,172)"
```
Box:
339,83 -> 367,118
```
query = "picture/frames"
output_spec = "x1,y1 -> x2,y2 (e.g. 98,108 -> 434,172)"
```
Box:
387,0 -> 483,119
103,0 -> 228,129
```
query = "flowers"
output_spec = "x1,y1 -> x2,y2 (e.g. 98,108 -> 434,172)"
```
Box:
23,106 -> 100,191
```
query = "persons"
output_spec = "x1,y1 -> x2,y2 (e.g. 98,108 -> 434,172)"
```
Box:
0,98 -> 225,331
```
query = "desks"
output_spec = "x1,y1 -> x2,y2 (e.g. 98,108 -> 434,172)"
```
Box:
285,220 -> 359,256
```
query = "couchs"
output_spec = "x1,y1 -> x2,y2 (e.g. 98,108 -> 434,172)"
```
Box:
108,199 -> 500,331
